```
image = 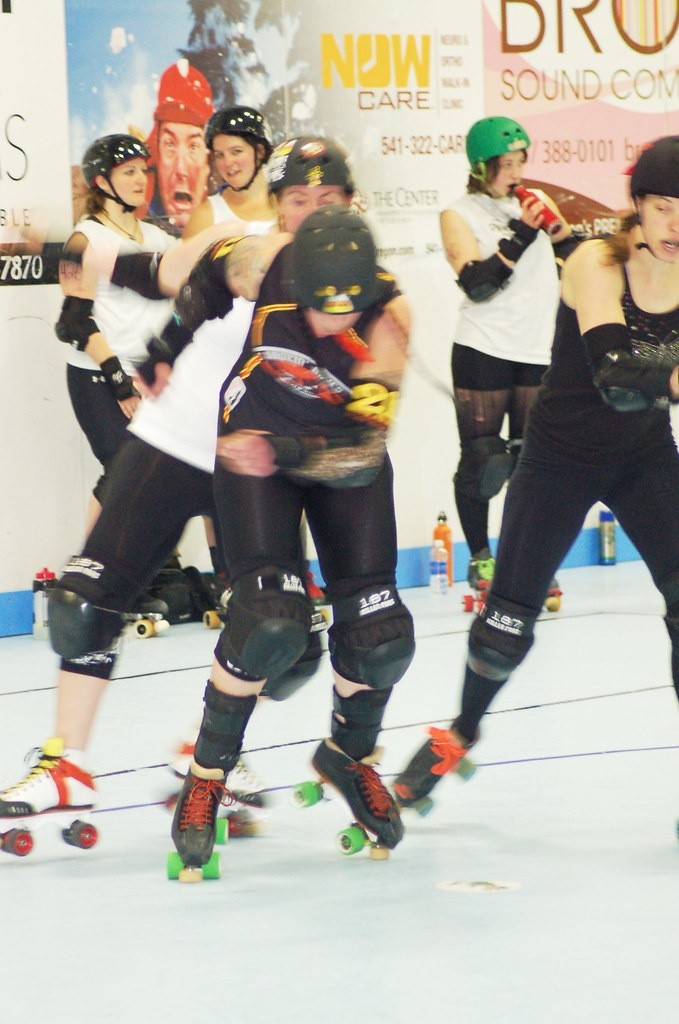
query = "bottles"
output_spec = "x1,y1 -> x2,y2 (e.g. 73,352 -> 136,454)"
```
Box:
598,508 -> 617,566
429,539 -> 448,596
32,567 -> 58,641
433,511 -> 453,587
509,182 -> 562,237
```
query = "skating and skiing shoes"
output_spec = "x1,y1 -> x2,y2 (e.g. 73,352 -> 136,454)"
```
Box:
121,593 -> 169,639
293,741 -> 405,862
165,768 -> 227,882
0,736 -> 98,856
542,580 -> 563,611
395,720 -> 475,818
203,579 -> 233,629
165,731 -> 261,838
461,557 -> 497,612
305,573 -> 324,608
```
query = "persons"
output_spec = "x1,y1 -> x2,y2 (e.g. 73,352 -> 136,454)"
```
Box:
1,106 -> 578,633
142,59 -> 225,225
0,138 -> 354,859
134,209 -> 417,884
390,138 -> 679,836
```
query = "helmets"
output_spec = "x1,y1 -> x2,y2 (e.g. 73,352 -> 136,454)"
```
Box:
83,135 -> 149,185
153,64 -> 213,130
465,117 -> 532,174
629,137 -> 679,198
203,106 -> 273,161
269,138 -> 354,191
291,202 -> 381,314
